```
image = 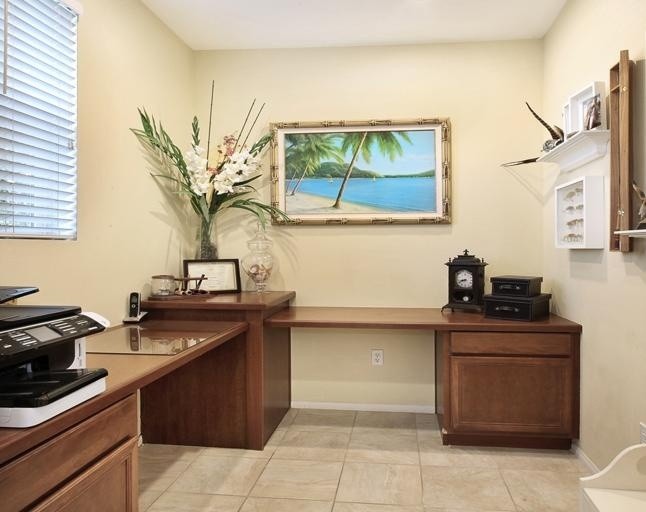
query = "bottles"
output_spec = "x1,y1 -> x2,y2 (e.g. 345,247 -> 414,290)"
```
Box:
241,220 -> 275,297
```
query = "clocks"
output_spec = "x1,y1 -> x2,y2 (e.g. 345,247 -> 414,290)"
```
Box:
442,248 -> 486,315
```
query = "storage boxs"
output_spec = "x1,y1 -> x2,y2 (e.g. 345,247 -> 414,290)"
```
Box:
489,273 -> 544,298
483,293 -> 557,318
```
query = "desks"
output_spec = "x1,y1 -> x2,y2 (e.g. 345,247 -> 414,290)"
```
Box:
0,289 -> 583,512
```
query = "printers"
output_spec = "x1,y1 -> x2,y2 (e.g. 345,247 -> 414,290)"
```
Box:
0,285 -> 110,429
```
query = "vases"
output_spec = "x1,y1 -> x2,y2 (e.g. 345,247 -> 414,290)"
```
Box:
195,219 -> 217,259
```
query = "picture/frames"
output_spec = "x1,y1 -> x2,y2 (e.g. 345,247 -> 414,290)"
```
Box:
267,116 -> 453,226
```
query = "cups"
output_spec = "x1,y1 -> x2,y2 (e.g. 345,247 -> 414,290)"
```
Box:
149,273 -> 177,297
147,338 -> 178,355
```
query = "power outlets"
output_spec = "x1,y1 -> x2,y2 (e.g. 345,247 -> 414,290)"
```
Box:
373,349 -> 381,367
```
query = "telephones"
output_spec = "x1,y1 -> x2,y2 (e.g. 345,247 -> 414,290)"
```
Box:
123,291 -> 148,324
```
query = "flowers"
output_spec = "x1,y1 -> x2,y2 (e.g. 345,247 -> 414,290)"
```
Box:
128,80 -> 291,243
